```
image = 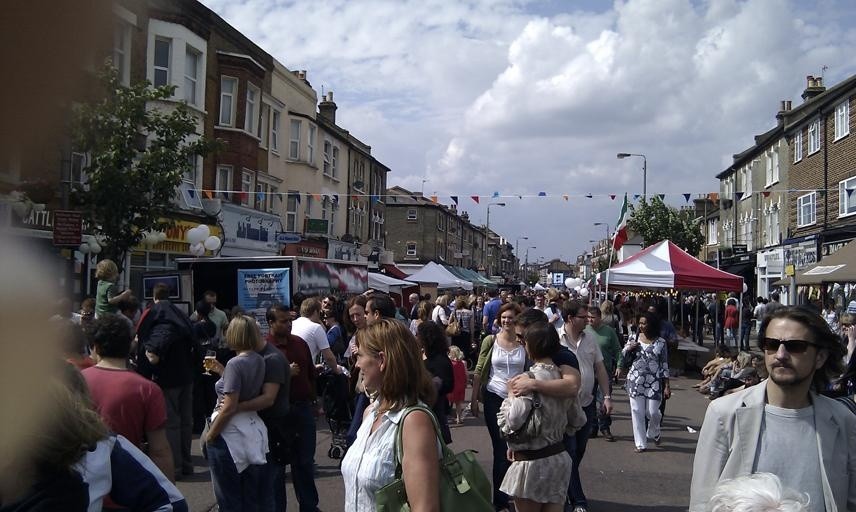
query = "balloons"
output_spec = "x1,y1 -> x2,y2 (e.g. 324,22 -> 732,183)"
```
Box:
185,223 -> 220,257
78,226 -> 110,254
135,226 -> 165,245
565,278 -> 588,297
9,190 -> 45,218
743,283 -> 747,294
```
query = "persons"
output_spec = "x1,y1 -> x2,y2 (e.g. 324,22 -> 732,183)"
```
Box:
339,295 -> 366,468
533,293 -> 546,311
78,314 -> 174,480
408,293 -> 419,319
425,293 -> 435,319
583,306 -> 625,442
839,313 -> 855,365
0,359 -> 190,512
190,289 -> 228,351
317,305 -> 353,440
754,297 -> 767,336
497,321 -> 587,511
621,311 -> 671,454
544,288 -> 569,327
449,296 -> 475,358
448,345 -> 466,424
821,298 -> 837,327
80,297 -> 95,314
606,291 -> 670,305
51,296 -> 81,352
555,298 -> 612,509
742,300 -> 755,351
692,344 -> 765,400
598,299 -> 620,335
690,297 -> 708,346
291,298 -> 343,375
137,281 -> 199,343
202,315 -> 270,510
193,301 -> 216,439
513,309 -> 580,398
320,296 -> 334,321
431,295 -> 453,326
482,287 -> 503,335
340,318 -> 443,511
472,296 -> 486,335
265,304 -> 319,512
499,289 -> 532,310
203,321 -> 293,511
416,321 -> 453,446
409,300 -> 431,336
724,300 -> 739,351
688,305 -> 856,511
144,323 -> 195,479
94,259 -> 136,355
765,293 -> 785,317
636,303 -> 678,433
709,293 -> 725,349
471,302 -> 527,512
363,292 -> 397,326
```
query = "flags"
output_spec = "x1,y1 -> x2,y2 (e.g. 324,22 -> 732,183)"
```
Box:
174,190 -> 852,205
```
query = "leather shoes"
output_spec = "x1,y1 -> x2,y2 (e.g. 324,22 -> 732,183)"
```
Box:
634,448 -> 648,452
654,434 -> 662,445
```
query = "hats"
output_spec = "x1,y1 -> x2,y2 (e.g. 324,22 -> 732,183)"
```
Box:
737,367 -> 757,380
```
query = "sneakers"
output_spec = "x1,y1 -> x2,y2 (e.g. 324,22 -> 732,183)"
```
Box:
691,384 -> 698,388
454,415 -> 464,422
699,389 -> 709,394
588,432 -> 599,438
601,429 -> 613,441
697,383 -> 702,387
709,392 -> 717,400
699,384 -> 709,389
447,415 -> 452,420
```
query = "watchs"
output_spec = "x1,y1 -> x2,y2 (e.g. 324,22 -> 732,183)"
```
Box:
603,395 -> 612,399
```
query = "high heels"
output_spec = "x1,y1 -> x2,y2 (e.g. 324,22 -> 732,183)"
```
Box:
456,420 -> 461,424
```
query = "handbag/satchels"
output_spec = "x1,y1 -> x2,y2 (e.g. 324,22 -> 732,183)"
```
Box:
624,332 -> 640,368
471,334 -> 497,402
498,373 -> 543,444
446,310 -> 461,337
607,314 -> 620,333
376,405 -> 494,511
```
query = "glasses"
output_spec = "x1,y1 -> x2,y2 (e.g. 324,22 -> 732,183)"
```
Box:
743,377 -> 752,380
575,315 -> 587,319
207,302 -> 217,307
841,322 -> 853,327
757,337 -> 817,355
322,316 -> 330,320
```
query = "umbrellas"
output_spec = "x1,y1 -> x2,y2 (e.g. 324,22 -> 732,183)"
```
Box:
772,237 -> 856,284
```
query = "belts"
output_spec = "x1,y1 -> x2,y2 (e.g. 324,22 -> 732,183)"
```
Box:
507,440 -> 566,461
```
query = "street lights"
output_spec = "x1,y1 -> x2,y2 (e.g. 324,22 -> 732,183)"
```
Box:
594,222 -> 609,261
590,240 -> 600,246
485,203 -> 505,268
515,236 -> 528,279
525,247 -> 536,280
617,153 -> 647,205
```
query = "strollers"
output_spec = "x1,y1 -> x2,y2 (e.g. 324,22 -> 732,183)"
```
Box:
315,362 -> 358,460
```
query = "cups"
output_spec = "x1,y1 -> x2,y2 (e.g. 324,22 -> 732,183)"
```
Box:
203,350 -> 216,375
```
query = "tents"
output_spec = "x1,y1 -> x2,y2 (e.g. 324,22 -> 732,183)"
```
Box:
588,238 -> 743,351
404,261 -> 497,294
